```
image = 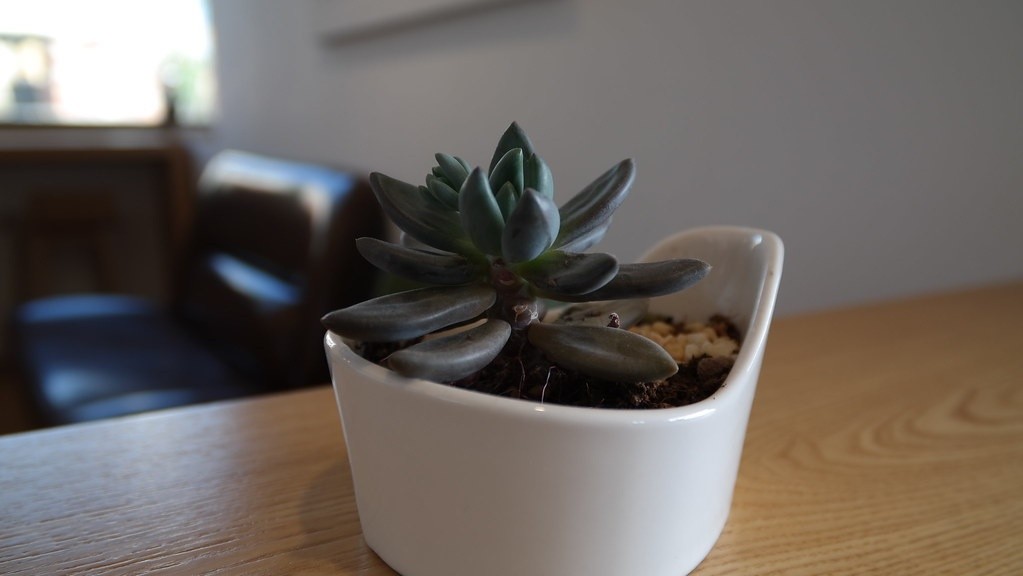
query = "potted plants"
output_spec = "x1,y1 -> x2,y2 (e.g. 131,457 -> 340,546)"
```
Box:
321,117 -> 786,576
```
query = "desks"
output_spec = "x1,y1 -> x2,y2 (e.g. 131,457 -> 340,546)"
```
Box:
1,281 -> 1023,574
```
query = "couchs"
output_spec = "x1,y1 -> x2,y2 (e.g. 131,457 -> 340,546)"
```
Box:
16,144 -> 368,425
